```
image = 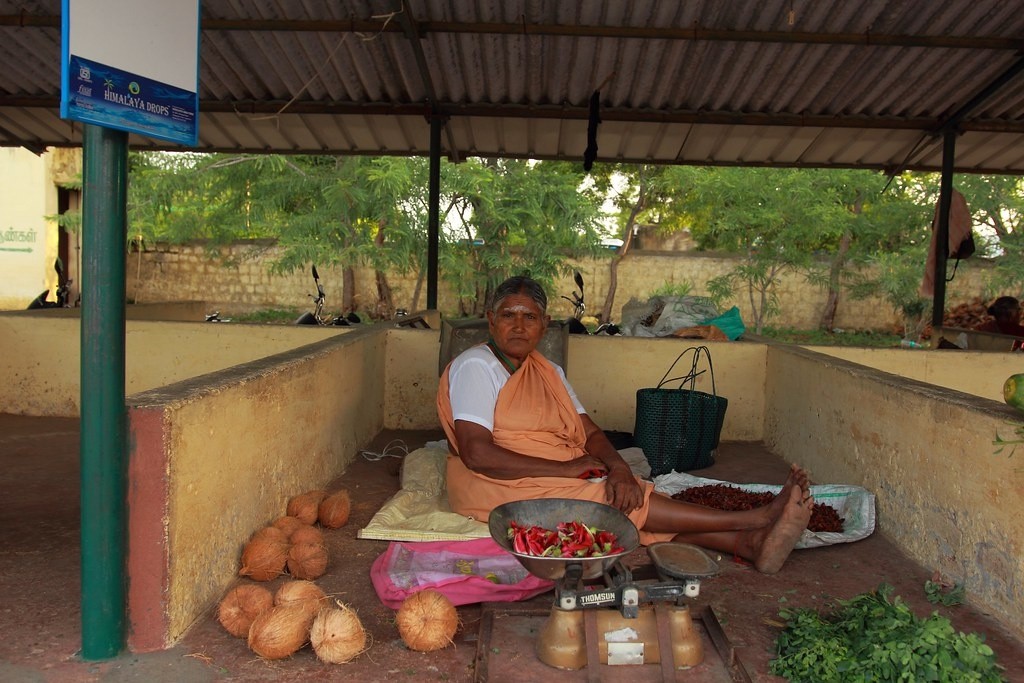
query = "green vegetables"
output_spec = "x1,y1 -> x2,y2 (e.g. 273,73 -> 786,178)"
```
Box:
766,583 -> 1009,683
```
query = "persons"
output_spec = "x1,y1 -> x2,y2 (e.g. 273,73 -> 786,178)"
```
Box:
434,277 -> 813,575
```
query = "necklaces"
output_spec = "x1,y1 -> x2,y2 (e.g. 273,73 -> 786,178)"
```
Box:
489,336 -> 517,372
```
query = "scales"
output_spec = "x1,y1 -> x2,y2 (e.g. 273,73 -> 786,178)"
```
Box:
487,497 -> 725,671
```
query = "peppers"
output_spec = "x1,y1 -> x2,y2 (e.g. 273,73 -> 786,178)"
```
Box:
506,519 -> 625,558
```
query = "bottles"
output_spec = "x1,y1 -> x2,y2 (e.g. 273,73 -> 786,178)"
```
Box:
901,339 -> 922,349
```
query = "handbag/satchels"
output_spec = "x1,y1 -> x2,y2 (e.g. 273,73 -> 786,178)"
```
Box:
634,346 -> 728,477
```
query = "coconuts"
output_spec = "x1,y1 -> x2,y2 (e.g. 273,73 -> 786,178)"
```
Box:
218,490 -> 365,665
394,590 -> 459,649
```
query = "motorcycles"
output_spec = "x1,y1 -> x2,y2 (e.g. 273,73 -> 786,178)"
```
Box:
560,269 -> 626,336
296,264 -> 361,326
